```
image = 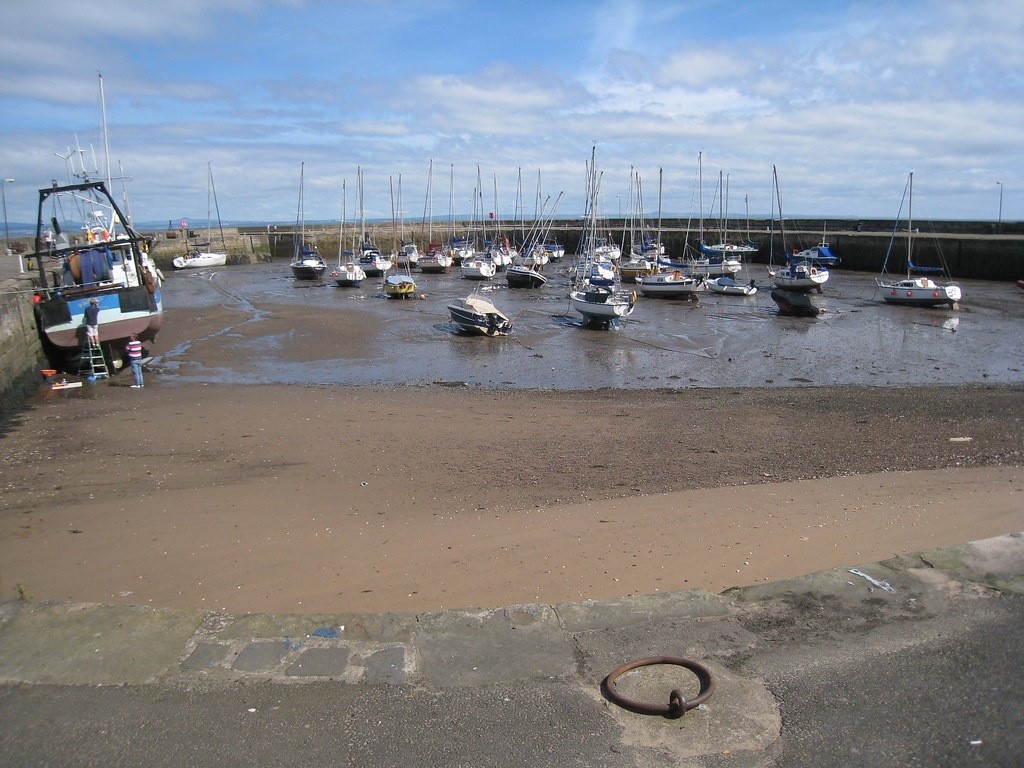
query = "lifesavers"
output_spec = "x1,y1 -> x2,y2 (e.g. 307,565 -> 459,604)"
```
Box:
545,252 -> 549,257
906,291 -> 912,297
633,290 -> 636,300
371,261 -> 376,266
891,290 -> 896,295
810,268 -> 818,275
610,264 -> 615,270
785,261 -> 792,266
934,291 -> 939,297
792,249 -> 799,254
89,227 -> 109,243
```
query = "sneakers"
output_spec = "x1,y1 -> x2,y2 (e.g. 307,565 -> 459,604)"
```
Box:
130,385 -> 144,388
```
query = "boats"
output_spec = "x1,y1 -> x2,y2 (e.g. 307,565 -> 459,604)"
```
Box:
172,249 -> 227,268
569,287 -> 635,327
446,297 -> 514,337
793,246 -> 840,266
702,276 -> 757,295
24,180 -> 164,355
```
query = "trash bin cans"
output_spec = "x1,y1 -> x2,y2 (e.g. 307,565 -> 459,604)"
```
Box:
6,249 -> 12,256
190,231 -> 194,238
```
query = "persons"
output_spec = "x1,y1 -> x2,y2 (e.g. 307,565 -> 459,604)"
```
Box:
85,298 -> 100,349
44,227 -> 54,252
125,333 -> 144,388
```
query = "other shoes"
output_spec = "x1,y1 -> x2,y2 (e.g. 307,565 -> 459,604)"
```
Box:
92,344 -> 99,350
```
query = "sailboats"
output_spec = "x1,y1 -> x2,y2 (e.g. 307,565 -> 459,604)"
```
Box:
686,152 -> 742,278
764,164 -> 829,291
874,171 -> 963,308
290,142 -> 702,300
700,170 -> 759,262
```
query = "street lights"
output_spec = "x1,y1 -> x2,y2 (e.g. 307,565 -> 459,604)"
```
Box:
996,180 -> 1003,234
1,178 -> 16,249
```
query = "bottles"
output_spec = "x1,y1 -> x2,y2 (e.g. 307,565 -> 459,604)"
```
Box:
62,379 -> 66,386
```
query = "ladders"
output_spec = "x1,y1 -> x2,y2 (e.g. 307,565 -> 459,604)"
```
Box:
77,332 -> 110,379
